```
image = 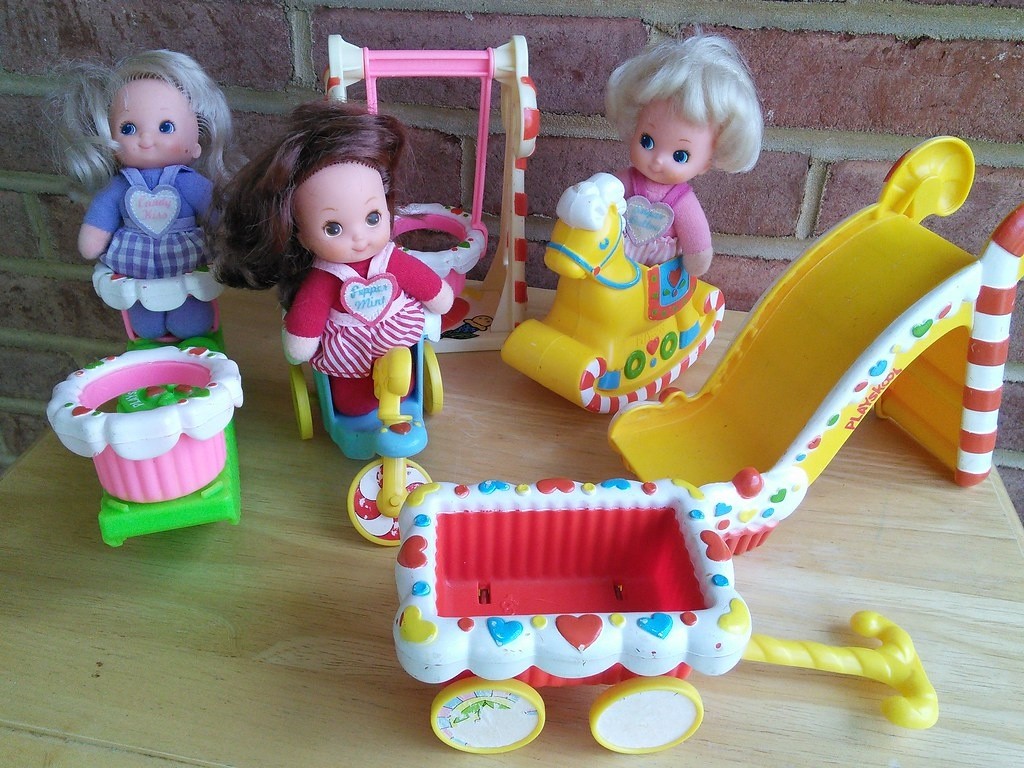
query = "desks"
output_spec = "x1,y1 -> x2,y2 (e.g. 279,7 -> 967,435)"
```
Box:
0,286 -> 1024,768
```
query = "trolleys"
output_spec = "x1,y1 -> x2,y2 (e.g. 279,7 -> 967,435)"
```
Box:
394,477 -> 941,754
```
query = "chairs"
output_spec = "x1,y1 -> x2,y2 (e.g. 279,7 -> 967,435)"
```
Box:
121,295 -> 221,344
310,329 -> 428,430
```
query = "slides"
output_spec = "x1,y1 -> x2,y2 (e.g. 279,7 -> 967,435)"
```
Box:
606,206 -> 982,489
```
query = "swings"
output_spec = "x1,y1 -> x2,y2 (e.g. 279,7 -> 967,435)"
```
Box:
363,46 -> 496,299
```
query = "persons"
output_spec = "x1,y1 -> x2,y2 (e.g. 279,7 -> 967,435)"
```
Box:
52,49 -> 244,341
605,34 -> 763,277
211,99 -> 456,417
